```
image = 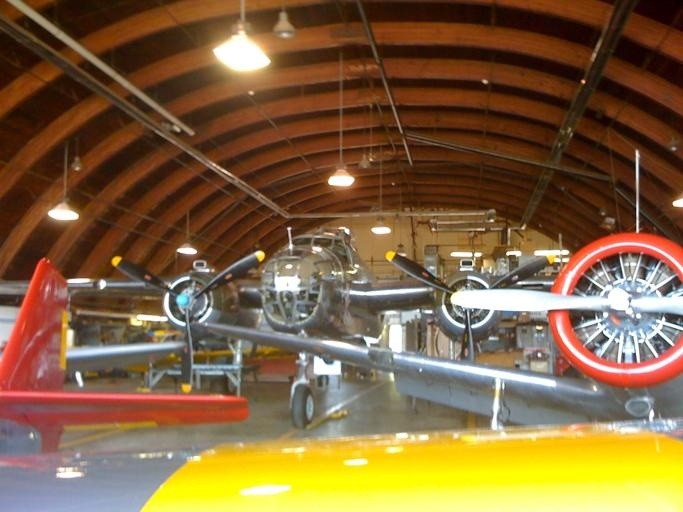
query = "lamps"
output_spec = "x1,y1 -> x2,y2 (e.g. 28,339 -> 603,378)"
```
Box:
326,27 -> 394,237
271,0 -> 296,38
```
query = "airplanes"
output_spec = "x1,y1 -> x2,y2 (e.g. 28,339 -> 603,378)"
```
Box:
0,224 -> 683,441
